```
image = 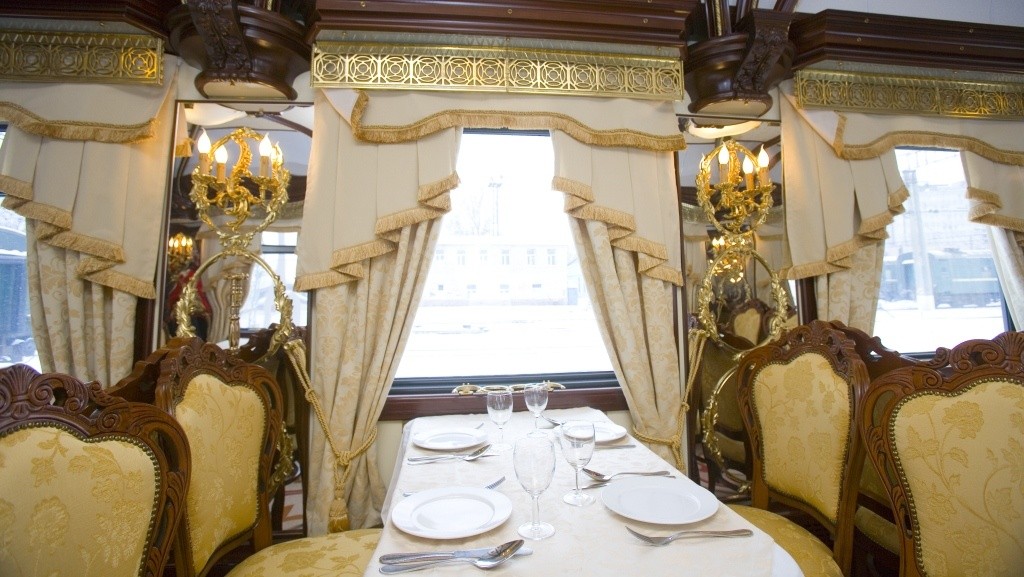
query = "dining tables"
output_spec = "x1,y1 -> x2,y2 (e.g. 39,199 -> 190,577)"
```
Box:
362,406 -> 806,577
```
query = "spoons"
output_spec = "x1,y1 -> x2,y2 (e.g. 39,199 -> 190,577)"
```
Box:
406,443 -> 492,465
380,539 -> 524,574
582,467 -> 670,482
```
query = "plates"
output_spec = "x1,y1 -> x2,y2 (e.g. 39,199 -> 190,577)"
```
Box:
563,423 -> 626,443
391,487 -> 513,539
413,430 -> 488,450
600,475 -> 719,525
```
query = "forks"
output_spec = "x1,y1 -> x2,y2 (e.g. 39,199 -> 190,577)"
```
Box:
403,476 -> 505,497
625,526 -> 753,546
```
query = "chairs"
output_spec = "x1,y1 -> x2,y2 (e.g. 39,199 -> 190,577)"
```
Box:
824,319 -> 953,577
159,333 -> 386,577
0,364 -> 193,577
861,330 -> 1024,577
722,317 -> 874,577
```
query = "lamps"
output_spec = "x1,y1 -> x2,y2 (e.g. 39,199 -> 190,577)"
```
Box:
174,121 -> 293,357
692,136 -> 788,348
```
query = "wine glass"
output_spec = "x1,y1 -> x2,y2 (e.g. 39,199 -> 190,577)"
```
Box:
523,384 -> 547,434
559,423 -> 596,506
514,437 -> 557,541
487,391 -> 513,454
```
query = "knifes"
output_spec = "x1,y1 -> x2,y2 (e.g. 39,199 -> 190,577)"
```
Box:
408,454 -> 501,461
380,548 -> 533,564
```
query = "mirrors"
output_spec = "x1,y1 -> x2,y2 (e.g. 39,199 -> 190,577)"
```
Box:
155,94 -> 317,371
672,107 -> 806,384
